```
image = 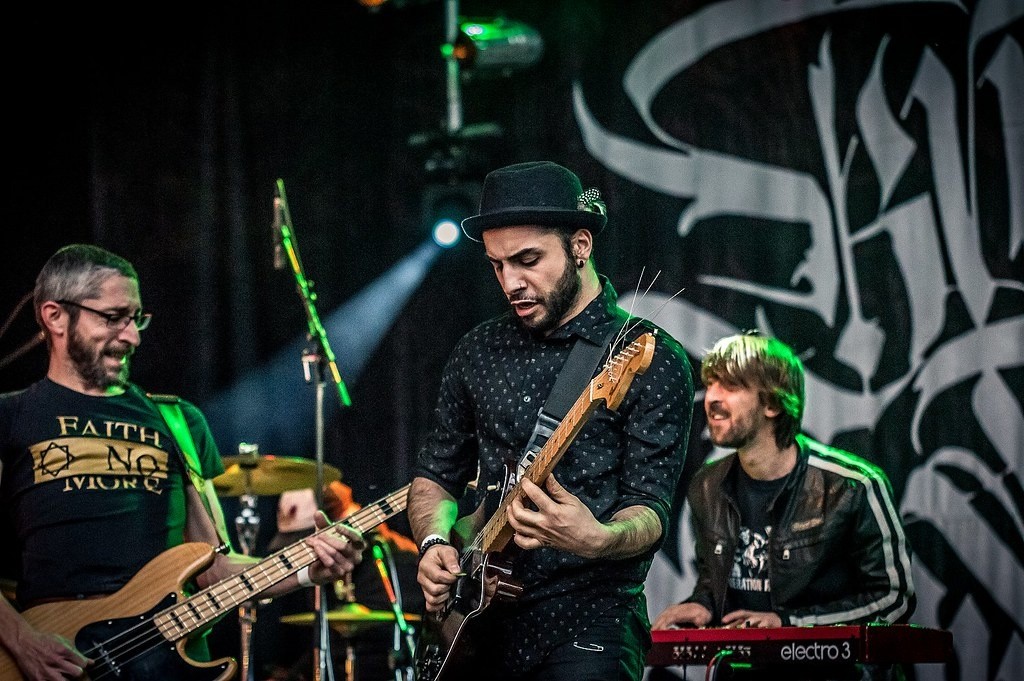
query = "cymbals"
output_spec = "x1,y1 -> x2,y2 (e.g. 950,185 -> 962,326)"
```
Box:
212,454 -> 339,493
281,605 -> 423,637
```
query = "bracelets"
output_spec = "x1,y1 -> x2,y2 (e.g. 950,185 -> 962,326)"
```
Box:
297,567 -> 315,590
418,537 -> 450,562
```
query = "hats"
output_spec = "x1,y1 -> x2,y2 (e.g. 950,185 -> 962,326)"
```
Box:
461,160 -> 605,243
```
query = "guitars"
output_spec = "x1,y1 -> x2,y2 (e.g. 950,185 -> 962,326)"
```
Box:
413,264 -> 693,681
0,464 -> 480,680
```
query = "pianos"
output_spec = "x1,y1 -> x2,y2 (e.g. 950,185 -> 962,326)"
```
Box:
645,615 -> 952,667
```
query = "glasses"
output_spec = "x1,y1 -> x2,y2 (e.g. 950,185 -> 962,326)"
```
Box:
51,299 -> 153,332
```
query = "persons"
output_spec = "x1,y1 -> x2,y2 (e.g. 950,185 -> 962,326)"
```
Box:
0,244 -> 372,680
406,161 -> 697,681
269,481 -> 420,681
650,329 -> 917,681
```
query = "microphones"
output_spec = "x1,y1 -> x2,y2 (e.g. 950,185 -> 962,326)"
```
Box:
271,196 -> 283,268
